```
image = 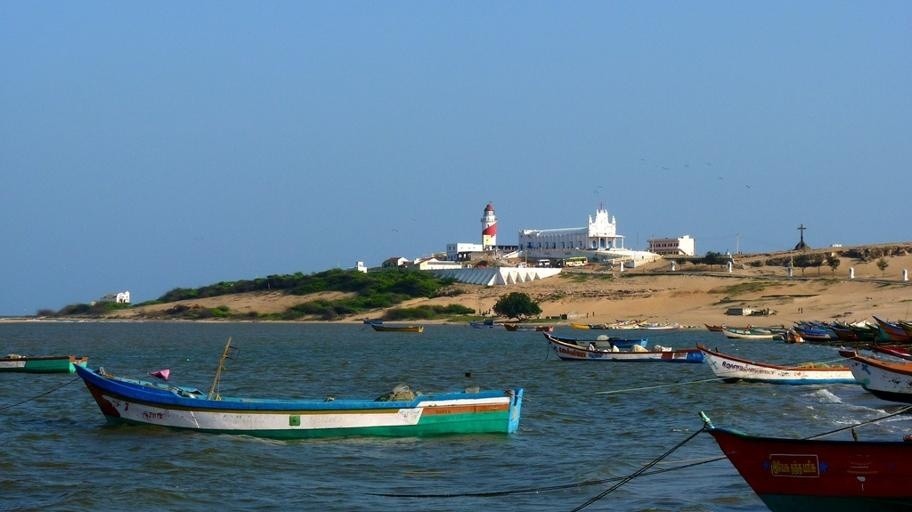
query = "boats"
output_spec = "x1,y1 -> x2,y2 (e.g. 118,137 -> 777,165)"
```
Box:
364,319 -> 424,333
470,320 -> 554,332
838,349 -> 912,404
543,332 -> 706,363
570,320 -> 680,330
704,316 -> 912,361
72,362 -> 523,439
0,354 -> 88,373
696,345 -> 860,384
699,410 -> 912,512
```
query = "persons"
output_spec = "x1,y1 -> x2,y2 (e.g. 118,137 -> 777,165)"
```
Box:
10,351 -> 18,359
747,324 -> 751,329
720,323 -> 726,328
